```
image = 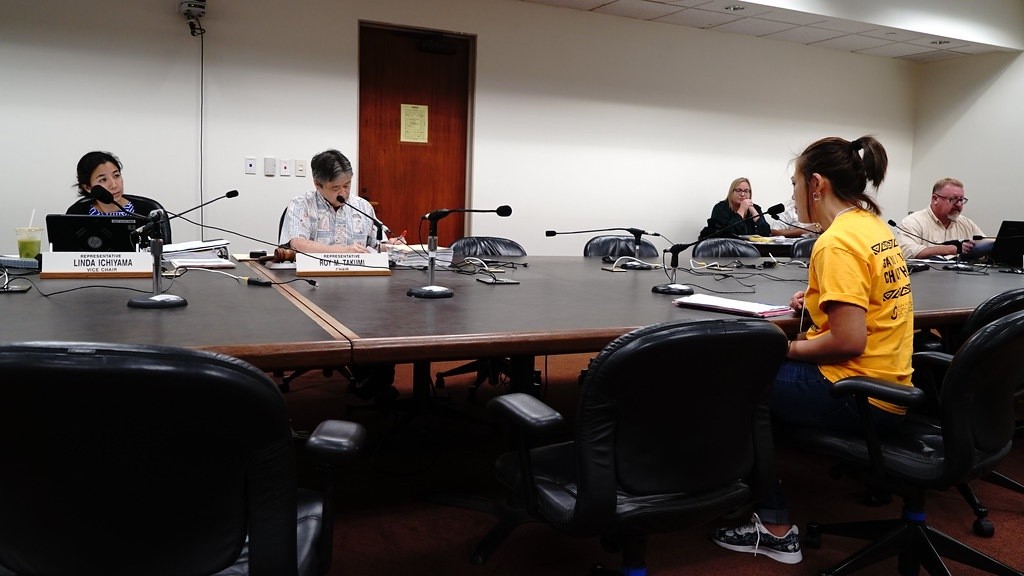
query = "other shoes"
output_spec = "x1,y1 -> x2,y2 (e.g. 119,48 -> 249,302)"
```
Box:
347,370 -> 399,400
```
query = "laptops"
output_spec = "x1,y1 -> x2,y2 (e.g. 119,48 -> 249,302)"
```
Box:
966,221 -> 1024,264
46,213 -> 149,253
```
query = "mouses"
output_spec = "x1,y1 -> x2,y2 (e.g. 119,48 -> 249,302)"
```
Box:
929,254 -> 948,261
602,255 -> 618,262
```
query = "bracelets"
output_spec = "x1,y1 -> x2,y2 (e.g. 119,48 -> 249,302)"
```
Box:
788,341 -> 792,350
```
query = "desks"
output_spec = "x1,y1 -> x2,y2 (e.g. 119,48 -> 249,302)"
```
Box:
745,238 -> 805,257
0,255 -> 352,373
244,256 -> 1023,363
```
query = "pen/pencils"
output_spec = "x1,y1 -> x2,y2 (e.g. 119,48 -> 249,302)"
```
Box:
769,253 -> 776,262
398,230 -> 407,239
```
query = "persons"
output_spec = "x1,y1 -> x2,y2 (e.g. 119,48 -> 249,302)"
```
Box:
707,177 -> 772,238
710,135 -> 915,564
770,200 -> 823,238
278,150 -> 407,253
65,151 -> 162,252
894,177 -> 988,259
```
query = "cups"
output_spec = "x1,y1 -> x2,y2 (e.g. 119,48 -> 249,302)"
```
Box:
16,227 -> 45,258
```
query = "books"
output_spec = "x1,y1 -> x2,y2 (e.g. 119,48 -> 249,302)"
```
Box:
162,239 -> 237,267
672,294 -> 796,318
387,244 -> 453,267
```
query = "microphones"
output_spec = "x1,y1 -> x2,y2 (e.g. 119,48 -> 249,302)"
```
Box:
422,205 -> 512,219
771,214 -> 823,234
91,185 -> 150,221
546,228 -> 660,236
336,195 -> 391,234
887,218 -> 970,245
972,235 -> 1024,240
664,204 -> 785,252
133,190 -> 239,235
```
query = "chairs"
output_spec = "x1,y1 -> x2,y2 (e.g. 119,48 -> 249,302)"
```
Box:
0,235 -> 1023,576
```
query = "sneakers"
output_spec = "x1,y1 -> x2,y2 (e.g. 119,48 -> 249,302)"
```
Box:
712,513 -> 802,564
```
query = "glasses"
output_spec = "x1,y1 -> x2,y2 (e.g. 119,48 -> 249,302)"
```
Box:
733,187 -> 750,193
935,194 -> 968,204
